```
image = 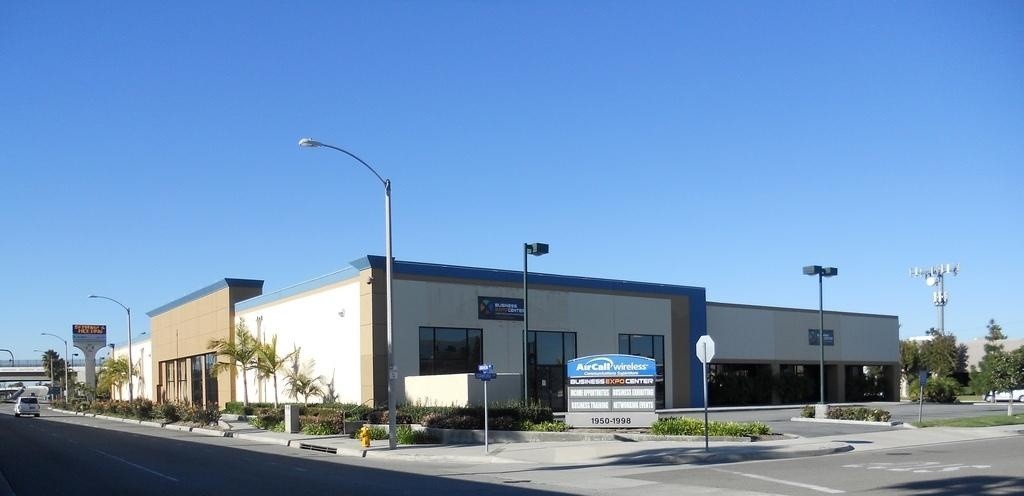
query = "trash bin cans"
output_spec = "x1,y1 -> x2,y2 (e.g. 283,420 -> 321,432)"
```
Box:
284,404 -> 299,434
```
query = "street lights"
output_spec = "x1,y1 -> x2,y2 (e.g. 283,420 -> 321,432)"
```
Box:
911,264 -> 960,335
90,296 -> 133,404
300,139 -> 396,450
523,242 -> 549,407
34,349 -> 53,386
803,265 -> 837,403
41,332 -> 68,409
72,353 -> 78,370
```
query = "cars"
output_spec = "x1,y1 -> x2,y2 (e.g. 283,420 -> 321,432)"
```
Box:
983,389 -> 1024,404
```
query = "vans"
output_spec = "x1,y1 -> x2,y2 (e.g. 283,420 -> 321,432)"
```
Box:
14,396 -> 40,418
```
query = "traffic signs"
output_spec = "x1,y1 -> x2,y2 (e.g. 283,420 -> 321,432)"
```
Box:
474,373 -> 495,381
477,365 -> 493,372
